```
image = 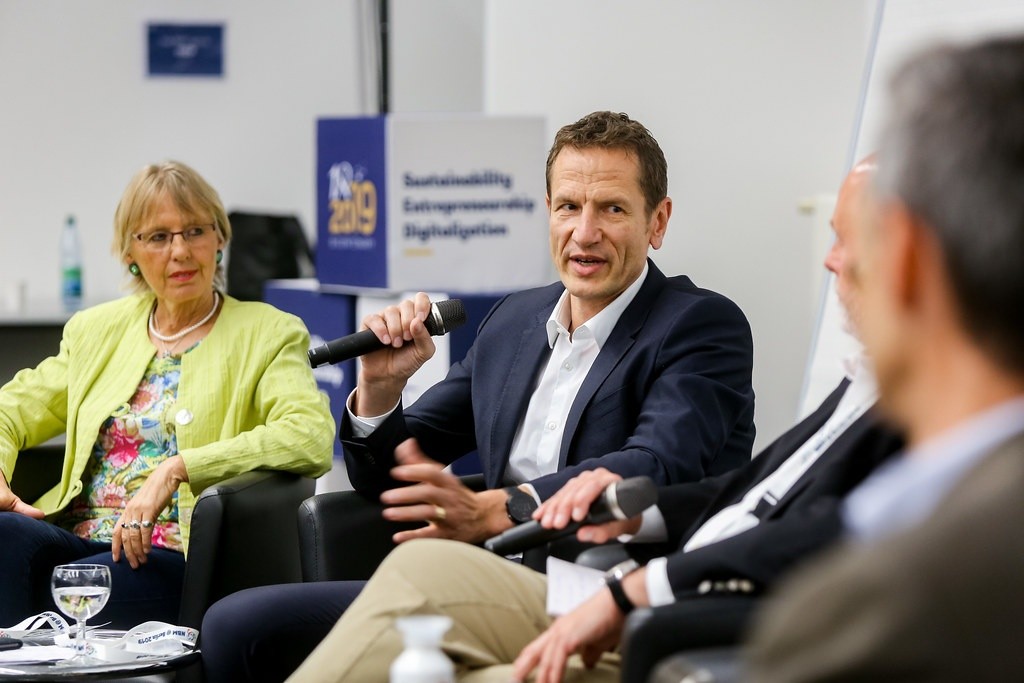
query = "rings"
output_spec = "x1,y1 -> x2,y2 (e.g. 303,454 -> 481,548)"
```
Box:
121,523 -> 129,528
427,505 -> 446,524
142,521 -> 155,527
129,520 -> 141,529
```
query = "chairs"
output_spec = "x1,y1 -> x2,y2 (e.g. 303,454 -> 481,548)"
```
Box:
0,444 -> 317,646
226,209 -> 309,303
296,472 -> 488,580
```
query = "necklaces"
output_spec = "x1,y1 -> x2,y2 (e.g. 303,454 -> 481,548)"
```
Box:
149,292 -> 219,358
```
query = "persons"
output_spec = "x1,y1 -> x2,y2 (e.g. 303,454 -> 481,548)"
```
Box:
750,34 -> 1024,683
0,160 -> 336,625
285,155 -> 907,683
202,111 -> 756,683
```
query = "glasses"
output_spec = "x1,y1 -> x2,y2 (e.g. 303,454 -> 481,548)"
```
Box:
132,223 -> 216,251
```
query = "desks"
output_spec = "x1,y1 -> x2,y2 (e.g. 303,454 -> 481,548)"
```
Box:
0,627 -> 202,683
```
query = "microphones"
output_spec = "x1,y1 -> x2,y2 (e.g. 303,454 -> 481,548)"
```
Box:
307,298 -> 467,369
486,475 -> 658,558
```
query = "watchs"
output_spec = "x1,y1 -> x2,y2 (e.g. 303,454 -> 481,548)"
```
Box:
605,560 -> 641,614
504,487 -> 539,525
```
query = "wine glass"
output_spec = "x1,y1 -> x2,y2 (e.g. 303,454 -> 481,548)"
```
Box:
51,563 -> 112,667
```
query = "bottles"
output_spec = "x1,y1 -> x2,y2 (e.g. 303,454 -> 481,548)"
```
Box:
390,615 -> 455,682
59,215 -> 84,312
